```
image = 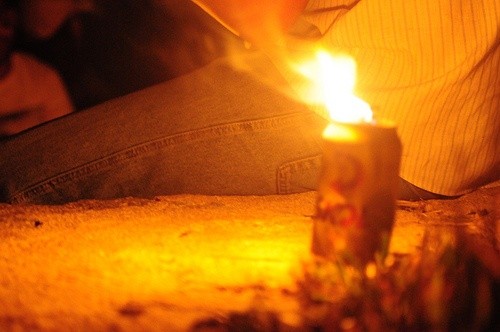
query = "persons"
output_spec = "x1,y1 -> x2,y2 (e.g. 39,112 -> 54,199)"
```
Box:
0,0 -> 111,139
0,0 -> 499,202
